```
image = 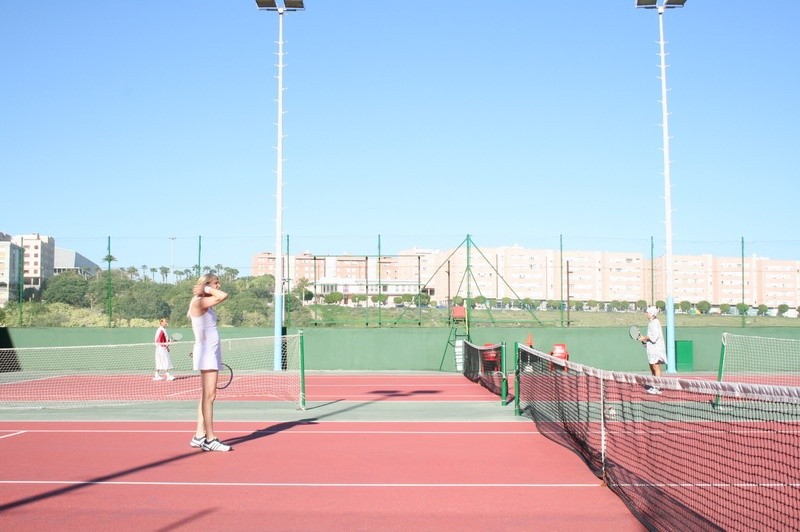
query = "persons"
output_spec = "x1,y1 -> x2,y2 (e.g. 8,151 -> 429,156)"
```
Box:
187,273 -> 232,451
153,317 -> 176,381
637,306 -> 668,394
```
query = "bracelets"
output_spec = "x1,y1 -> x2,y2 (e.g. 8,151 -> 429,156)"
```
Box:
204,286 -> 211,294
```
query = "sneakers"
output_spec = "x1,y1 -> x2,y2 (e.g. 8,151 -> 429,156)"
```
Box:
201,438 -> 232,451
646,387 -> 663,395
166,375 -> 175,380
152,375 -> 163,380
189,436 -> 207,448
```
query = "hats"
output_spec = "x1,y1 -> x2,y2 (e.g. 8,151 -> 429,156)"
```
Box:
646,306 -> 658,317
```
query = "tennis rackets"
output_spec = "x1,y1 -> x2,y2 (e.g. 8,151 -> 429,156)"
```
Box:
188,352 -> 232,389
169,333 -> 182,346
629,326 -> 645,343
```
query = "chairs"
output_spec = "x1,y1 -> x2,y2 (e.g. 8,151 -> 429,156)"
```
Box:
480,342 -> 503,373
548,342 -> 571,374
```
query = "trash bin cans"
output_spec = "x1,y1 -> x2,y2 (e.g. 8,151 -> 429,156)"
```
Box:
676,340 -> 692,372
